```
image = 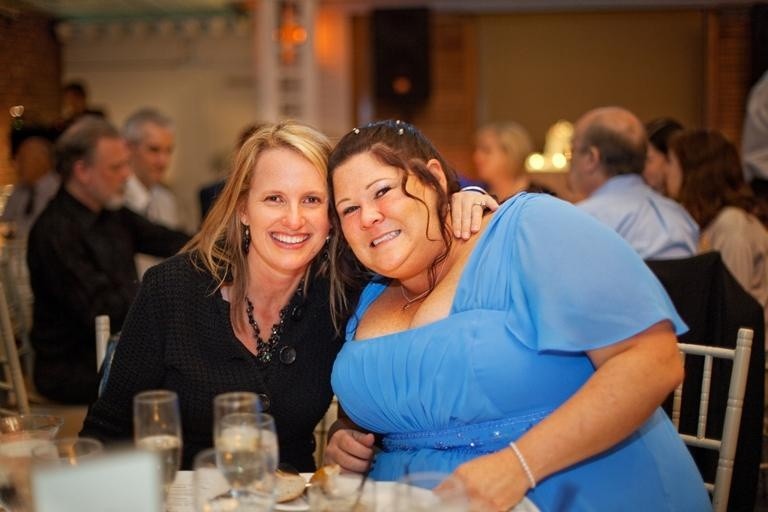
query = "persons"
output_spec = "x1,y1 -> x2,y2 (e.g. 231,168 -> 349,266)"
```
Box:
474,120 -> 557,203
322,120 -> 713,512
739,78 -> 768,190
84,123 -> 499,475
2,77 -> 196,416
568,106 -> 768,327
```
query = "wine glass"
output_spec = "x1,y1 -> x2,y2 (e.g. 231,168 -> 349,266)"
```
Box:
134,392 -> 182,502
214,393 -> 260,512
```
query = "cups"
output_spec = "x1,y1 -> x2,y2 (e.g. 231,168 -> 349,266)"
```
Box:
0,408 -> 467,511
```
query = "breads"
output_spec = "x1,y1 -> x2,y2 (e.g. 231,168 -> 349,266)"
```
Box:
274,472 -> 306,503
309,464 -> 341,485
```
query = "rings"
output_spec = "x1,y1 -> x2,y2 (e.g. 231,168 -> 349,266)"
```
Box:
472,201 -> 486,206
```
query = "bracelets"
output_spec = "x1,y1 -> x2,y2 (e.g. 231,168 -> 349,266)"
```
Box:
509,442 -> 536,489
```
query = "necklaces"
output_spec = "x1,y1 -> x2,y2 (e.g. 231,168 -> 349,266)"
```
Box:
244,238 -> 306,363
402,255 -> 449,309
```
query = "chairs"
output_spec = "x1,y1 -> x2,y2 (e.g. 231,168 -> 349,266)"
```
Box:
92,311 -> 123,376
0,267 -> 39,417
656,325 -> 757,511
